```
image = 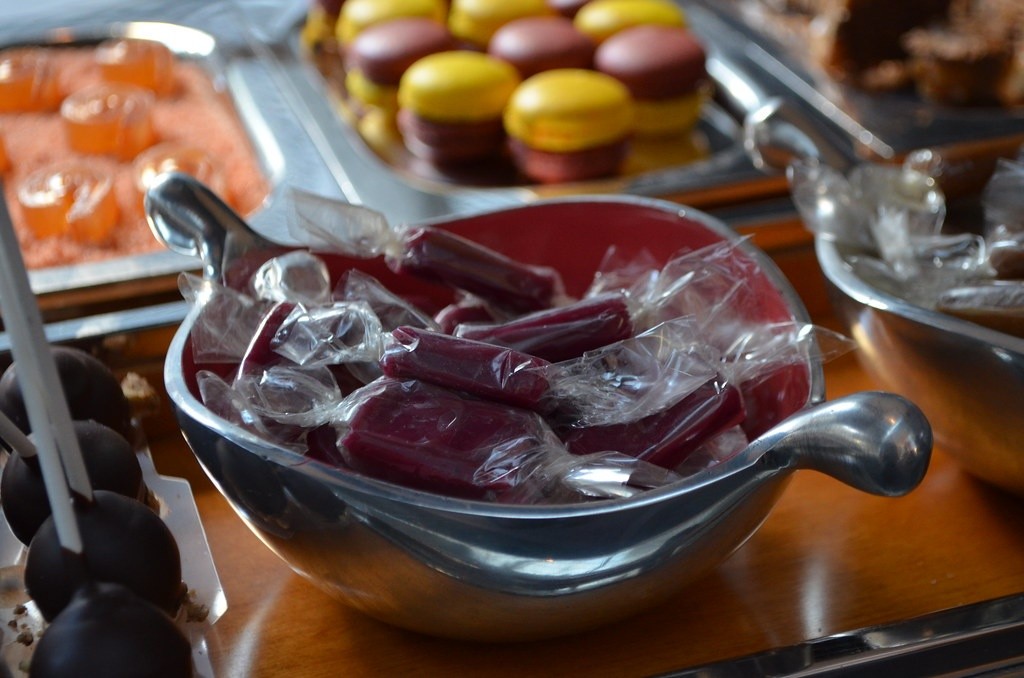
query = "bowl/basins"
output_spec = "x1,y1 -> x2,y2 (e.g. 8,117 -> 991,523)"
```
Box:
145,171 -> 933,649
812,134 -> 1024,501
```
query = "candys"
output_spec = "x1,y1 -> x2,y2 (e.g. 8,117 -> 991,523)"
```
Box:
0,40 -> 236,243
161,195 -> 842,513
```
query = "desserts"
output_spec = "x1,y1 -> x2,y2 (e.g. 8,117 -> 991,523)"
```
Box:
300,0 -> 707,183
